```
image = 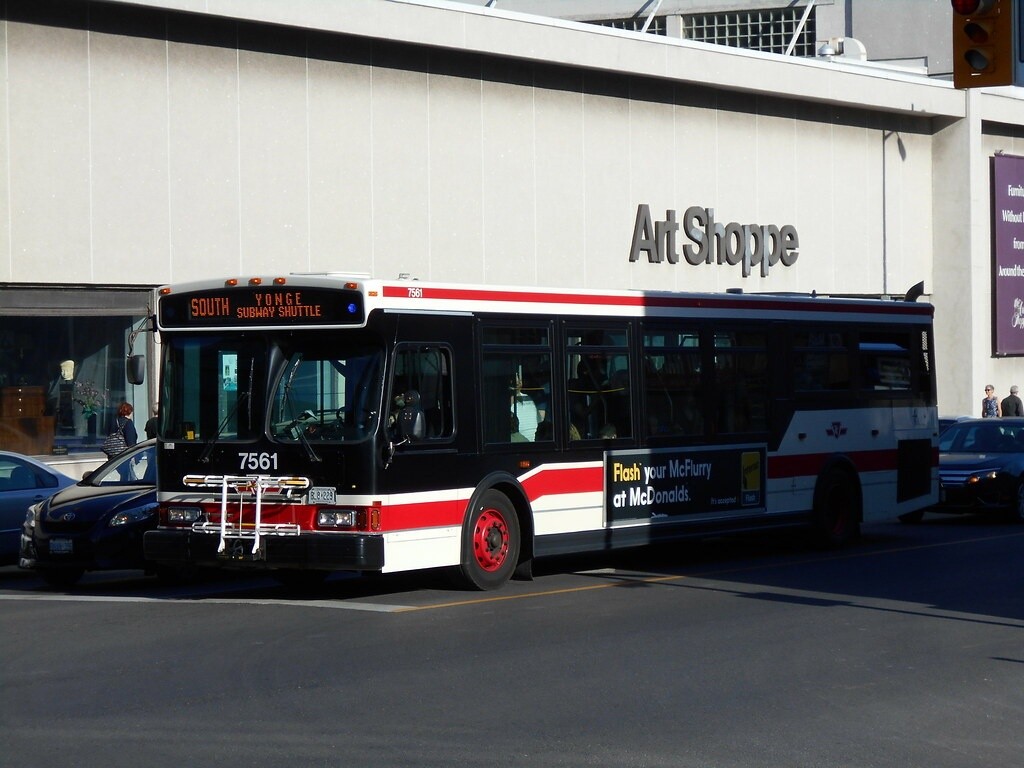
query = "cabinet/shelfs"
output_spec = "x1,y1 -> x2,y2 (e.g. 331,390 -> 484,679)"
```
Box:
0,385 -> 55,456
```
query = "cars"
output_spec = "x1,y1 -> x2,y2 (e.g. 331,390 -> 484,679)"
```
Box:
0,451 -> 84,555
938,414 -> 1024,524
18,432 -> 232,595
938,415 -> 973,437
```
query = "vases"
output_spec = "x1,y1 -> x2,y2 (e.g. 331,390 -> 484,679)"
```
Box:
88,414 -> 96,444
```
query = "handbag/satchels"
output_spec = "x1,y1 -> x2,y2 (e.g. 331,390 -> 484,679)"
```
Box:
101,419 -> 128,458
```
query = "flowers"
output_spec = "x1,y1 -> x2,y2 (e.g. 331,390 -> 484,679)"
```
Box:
72,379 -> 109,418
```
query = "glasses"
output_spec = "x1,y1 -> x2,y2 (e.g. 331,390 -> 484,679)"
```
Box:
985,389 -> 991,391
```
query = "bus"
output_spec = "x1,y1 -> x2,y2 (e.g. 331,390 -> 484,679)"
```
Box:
125,276 -> 950,591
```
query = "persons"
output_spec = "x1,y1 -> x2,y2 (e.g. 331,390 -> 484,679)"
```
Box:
510,373 -> 553,441
46,360 -> 89,438
982,385 -> 1002,418
100,402 -> 158,481
1001,385 -> 1024,417
